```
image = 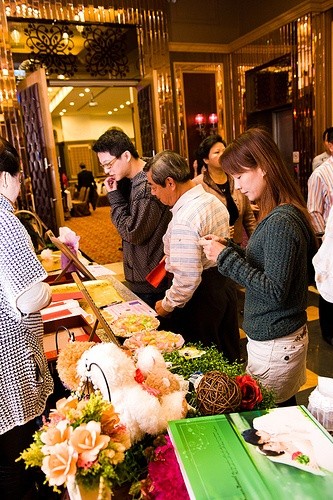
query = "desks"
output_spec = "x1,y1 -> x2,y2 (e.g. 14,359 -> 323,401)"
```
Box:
36,245 -> 278,500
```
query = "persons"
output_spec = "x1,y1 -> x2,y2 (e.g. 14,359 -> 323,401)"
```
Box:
77,163 -> 97,211
91,129 -> 173,310
197,128 -> 317,408
307,127 -> 333,347
141,150 -> 240,353
0,141 -> 67,500
240,406 -> 333,475
190,133 -> 258,363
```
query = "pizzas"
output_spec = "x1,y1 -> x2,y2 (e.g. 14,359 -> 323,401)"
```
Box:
111,314 -> 160,337
123,330 -> 184,354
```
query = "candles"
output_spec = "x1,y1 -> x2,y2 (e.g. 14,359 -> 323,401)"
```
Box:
210,114 -> 217,128
196,113 -> 203,128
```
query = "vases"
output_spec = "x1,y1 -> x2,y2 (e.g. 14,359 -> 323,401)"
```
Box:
61,242 -> 77,273
68,484 -> 111,500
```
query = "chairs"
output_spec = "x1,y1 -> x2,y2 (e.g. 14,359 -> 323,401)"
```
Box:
71,187 -> 90,216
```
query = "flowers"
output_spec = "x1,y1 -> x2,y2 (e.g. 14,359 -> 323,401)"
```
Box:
59,227 -> 80,250
15,392 -> 132,496
234,373 -> 263,410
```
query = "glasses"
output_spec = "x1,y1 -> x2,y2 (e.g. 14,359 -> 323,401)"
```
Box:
99,158 -> 117,169
